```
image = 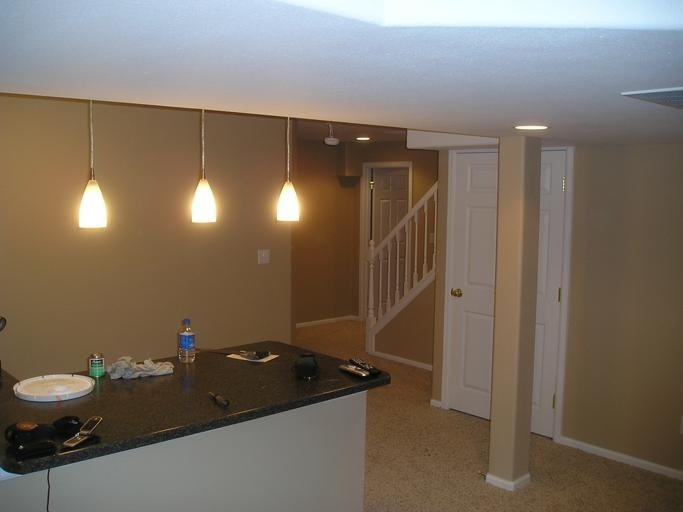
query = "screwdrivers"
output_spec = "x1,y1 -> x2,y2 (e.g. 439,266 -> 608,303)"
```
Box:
209,392 -> 229,409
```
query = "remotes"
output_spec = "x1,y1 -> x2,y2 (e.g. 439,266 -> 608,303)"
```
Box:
338,363 -> 370,379
351,356 -> 380,376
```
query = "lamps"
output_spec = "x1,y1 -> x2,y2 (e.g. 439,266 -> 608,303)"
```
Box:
337,174 -> 361,188
191,110 -> 217,223
79,101 -> 107,229
277,117 -> 300,222
324,123 -> 340,146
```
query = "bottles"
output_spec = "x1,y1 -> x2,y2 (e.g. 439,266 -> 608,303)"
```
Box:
176,319 -> 195,364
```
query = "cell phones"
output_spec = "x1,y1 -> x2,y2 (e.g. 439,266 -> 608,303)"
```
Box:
63,415 -> 102,448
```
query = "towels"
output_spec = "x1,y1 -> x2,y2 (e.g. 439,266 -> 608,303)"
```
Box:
106,355 -> 174,380
